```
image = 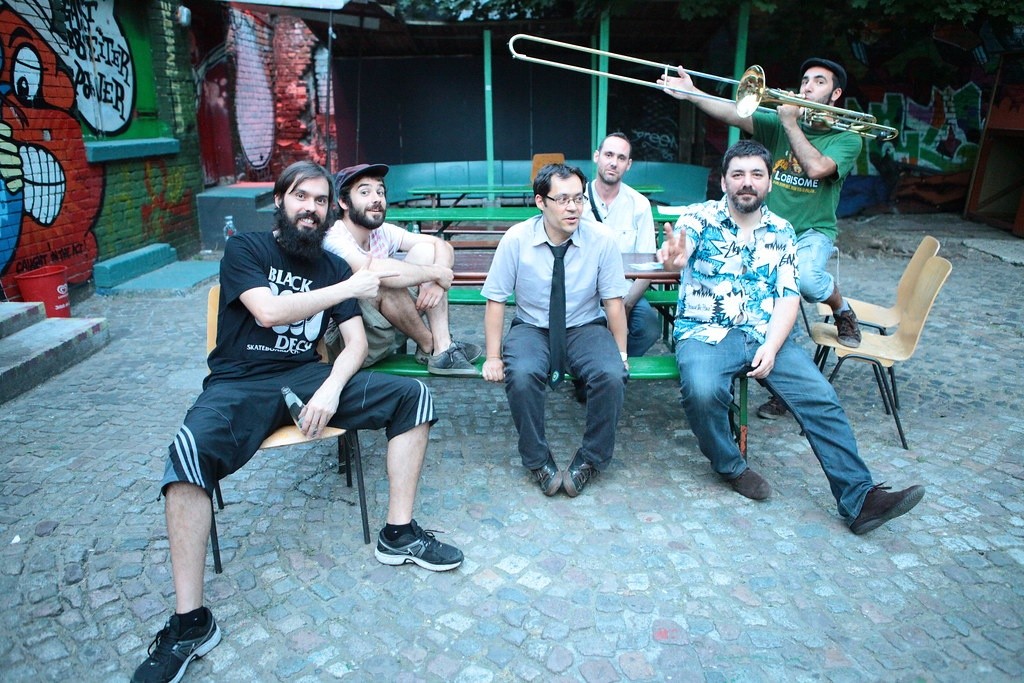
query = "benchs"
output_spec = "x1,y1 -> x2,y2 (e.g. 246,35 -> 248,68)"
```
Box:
355,185 -> 750,472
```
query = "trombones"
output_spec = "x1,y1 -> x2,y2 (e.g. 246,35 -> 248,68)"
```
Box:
507,34 -> 900,141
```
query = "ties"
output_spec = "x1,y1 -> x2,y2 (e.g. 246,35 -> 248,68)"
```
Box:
543,237 -> 574,391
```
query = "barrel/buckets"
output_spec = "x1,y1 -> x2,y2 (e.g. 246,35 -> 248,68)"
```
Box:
14,265 -> 72,318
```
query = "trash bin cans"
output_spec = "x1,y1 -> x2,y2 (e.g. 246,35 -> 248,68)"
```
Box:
14,265 -> 71,317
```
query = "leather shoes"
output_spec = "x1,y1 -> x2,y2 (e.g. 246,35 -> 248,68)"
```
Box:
850,480 -> 926,536
729,464 -> 773,502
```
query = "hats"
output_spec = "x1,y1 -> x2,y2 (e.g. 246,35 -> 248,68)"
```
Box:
799,57 -> 847,92
334,163 -> 389,199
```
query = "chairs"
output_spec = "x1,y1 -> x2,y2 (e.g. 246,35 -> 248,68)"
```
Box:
814,234 -> 941,411
532,153 -> 564,182
207,284 -> 370,574
790,255 -> 954,445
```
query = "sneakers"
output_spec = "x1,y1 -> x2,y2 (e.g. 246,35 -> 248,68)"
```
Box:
375,519 -> 465,572
562,444 -> 600,498
427,341 -> 482,376
757,396 -> 788,420
129,606 -> 222,683
832,301 -> 862,348
531,451 -> 562,498
414,333 -> 483,365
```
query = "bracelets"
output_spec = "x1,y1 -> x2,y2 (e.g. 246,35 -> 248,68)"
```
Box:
621,352 -> 627,361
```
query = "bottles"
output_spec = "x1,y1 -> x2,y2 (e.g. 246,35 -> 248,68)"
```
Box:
281,385 -> 319,437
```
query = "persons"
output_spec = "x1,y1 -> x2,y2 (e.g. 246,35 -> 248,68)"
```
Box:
132,161 -> 464,683
481,162 -> 631,497
658,140 -> 926,534
657,58 -> 862,349
322,163 -> 482,376
581,132 -> 662,357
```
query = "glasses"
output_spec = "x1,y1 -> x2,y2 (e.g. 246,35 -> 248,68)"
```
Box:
546,194 -> 589,206
